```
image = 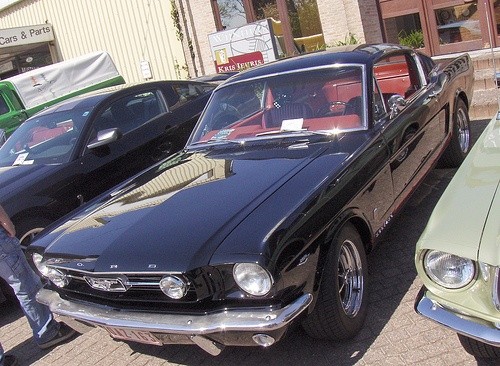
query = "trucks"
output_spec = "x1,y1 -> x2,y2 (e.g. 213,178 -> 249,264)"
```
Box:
0,50 -> 128,147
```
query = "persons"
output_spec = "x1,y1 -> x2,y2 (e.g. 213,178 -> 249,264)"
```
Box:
0,204 -> 75,366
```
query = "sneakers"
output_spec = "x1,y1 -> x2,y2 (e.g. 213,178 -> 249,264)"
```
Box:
36,320 -> 76,349
4,354 -> 17,366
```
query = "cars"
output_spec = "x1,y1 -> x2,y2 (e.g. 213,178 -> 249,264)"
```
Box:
0,70 -> 261,252
23,41 -> 476,357
412,71 -> 499,360
